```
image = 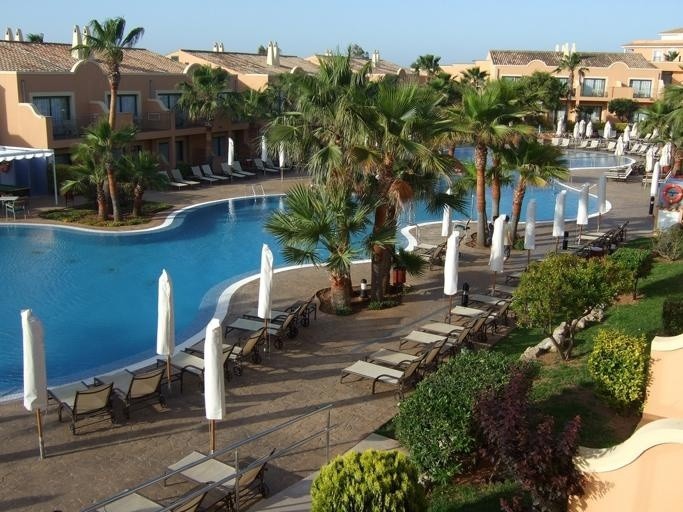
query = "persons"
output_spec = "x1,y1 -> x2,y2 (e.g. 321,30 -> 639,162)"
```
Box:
502,215 -> 512,264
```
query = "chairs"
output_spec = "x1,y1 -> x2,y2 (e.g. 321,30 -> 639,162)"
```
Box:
537,136 -> 674,190
4,199 -> 28,223
367,340 -> 448,386
93,480 -> 211,508
45,380 -> 121,437
340,350 -> 427,397
93,363 -> 167,419
401,291 -> 514,367
157,336 -> 241,394
185,325 -> 266,376
163,449 -> 274,509
157,156 -> 288,192
223,296 -> 316,352
406,233 -> 465,269
487,220 -> 630,296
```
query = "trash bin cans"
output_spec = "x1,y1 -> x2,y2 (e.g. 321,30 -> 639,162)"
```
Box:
393,266 -> 406,287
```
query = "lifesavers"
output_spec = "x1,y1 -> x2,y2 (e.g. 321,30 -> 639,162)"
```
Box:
663,183 -> 683,204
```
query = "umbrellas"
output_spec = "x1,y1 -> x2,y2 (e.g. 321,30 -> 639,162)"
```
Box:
155,269 -> 172,377
278,140 -> 286,182
256,243 -> 273,339
20,308 -> 46,458
442,233 -> 459,314
487,216 -> 504,292
523,198 -> 535,262
551,118 -> 677,247
227,137 -> 233,167
261,136 -> 267,174
204,318 -> 224,451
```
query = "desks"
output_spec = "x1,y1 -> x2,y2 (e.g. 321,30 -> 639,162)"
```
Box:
0,196 -> 18,212
